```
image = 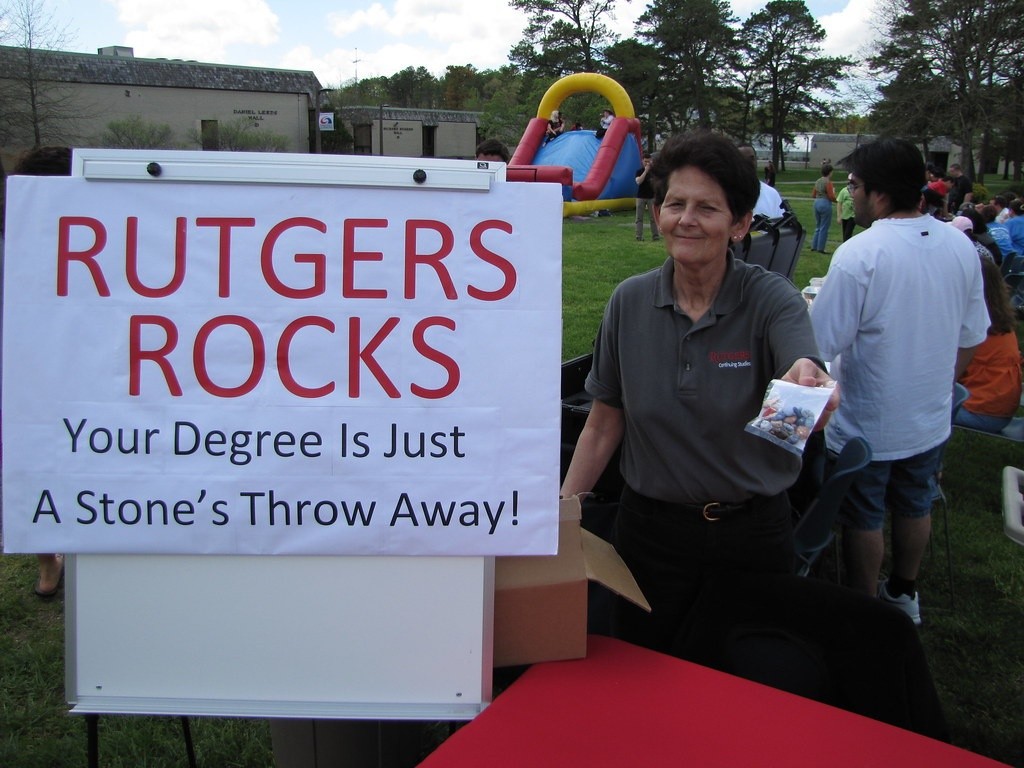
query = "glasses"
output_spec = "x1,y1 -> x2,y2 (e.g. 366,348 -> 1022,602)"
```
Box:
847,183 -> 865,194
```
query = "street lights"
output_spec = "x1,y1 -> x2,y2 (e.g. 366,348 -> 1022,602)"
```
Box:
379,103 -> 391,156
315,89 -> 335,154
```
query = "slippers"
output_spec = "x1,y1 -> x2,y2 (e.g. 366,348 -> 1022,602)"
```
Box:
35,554 -> 64,597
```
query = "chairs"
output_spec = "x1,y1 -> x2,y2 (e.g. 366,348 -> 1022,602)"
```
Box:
836,383 -> 971,616
792,437 -> 872,577
951,416 -> 1024,443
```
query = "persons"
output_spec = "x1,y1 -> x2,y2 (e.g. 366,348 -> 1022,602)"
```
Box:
635,153 -> 660,241
806,136 -> 991,626
836,173 -> 856,243
561,132 -> 841,672
475,139 -> 510,165
13,145 -> 72,595
570,120 -> 584,130
764,161 -> 777,187
596,108 -> 614,138
811,164 -> 838,253
951,255 -> 1023,434
919,162 -> 1024,267
736,144 -> 785,220
542,110 -> 565,147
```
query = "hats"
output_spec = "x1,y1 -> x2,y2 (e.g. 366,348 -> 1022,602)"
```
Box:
603,108 -> 613,115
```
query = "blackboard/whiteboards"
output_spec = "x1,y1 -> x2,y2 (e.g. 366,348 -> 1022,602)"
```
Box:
64,148 -> 504,721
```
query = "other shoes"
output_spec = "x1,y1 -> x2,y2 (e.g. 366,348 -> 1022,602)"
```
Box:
810,249 -> 817,251
818,250 -> 830,254
876,578 -> 923,628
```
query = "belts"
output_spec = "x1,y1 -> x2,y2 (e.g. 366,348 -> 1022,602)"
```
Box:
628,486 -> 784,521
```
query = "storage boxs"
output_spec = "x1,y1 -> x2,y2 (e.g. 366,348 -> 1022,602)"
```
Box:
497,497 -> 652,668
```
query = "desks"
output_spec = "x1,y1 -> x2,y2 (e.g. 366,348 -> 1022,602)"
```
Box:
415,635 -> 1014,768
